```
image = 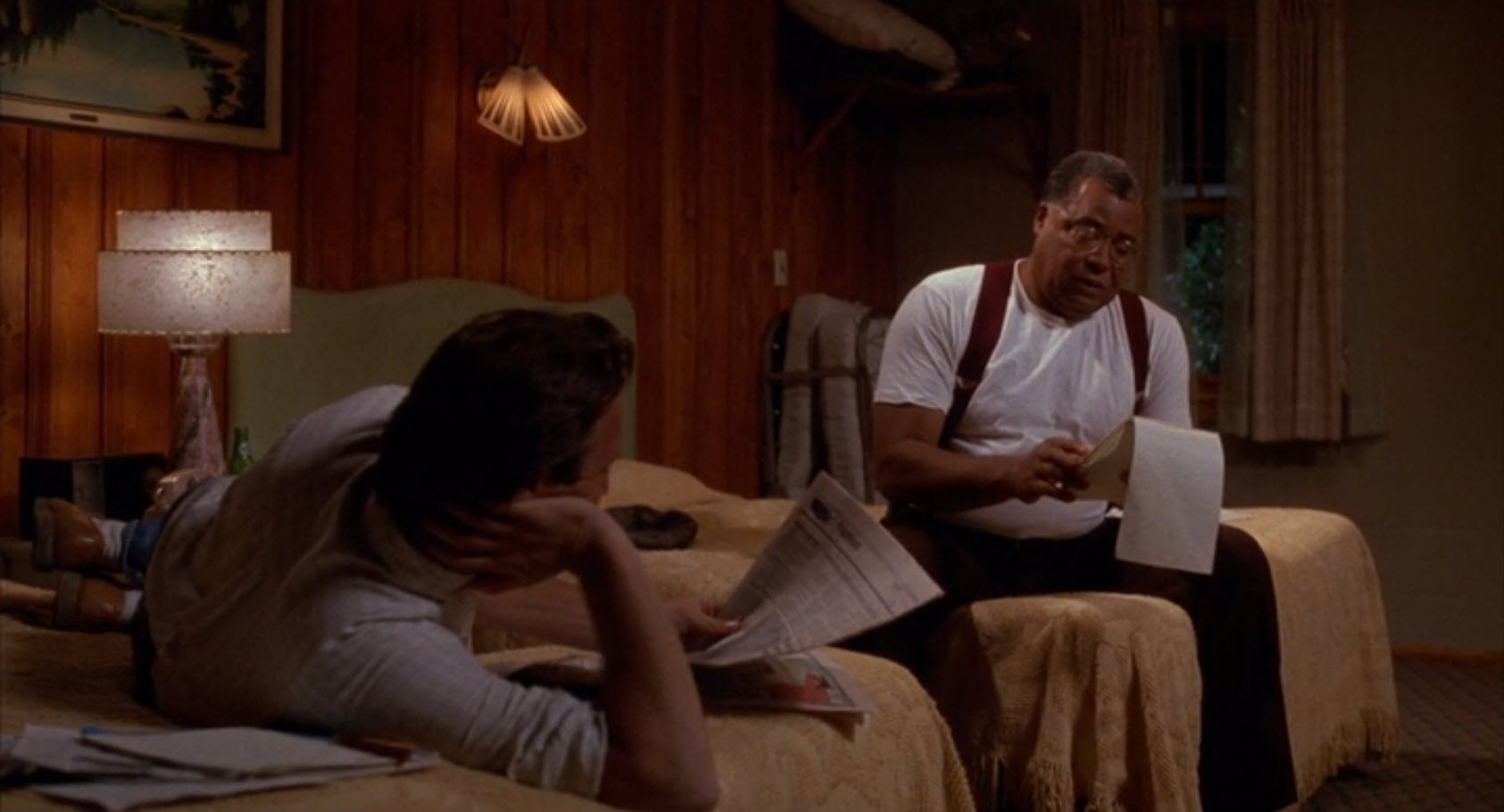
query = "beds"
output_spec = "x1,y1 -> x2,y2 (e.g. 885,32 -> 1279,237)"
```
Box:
442,541 -> 1201,812
593,458 -> 1399,810
0,533 -> 980,811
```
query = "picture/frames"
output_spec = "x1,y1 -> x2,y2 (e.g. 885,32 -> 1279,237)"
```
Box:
0,1 -> 282,150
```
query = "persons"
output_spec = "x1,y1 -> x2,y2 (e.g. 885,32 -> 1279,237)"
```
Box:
31,307 -> 759,812
853,151 -> 1297,812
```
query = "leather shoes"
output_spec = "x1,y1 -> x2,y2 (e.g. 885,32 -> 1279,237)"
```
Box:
48,570 -> 127,624
35,495 -> 108,569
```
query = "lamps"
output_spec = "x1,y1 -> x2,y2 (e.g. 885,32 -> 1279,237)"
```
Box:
477,60 -> 589,147
91,206 -> 296,482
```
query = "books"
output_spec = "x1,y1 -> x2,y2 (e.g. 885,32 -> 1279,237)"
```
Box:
1072,412 -> 1224,573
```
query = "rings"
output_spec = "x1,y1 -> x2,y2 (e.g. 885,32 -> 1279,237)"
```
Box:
1054,482 -> 1062,496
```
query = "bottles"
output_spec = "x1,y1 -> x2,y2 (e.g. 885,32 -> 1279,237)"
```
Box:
229,422 -> 252,475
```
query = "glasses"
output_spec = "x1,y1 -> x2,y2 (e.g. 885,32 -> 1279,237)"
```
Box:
1044,207 -> 1139,262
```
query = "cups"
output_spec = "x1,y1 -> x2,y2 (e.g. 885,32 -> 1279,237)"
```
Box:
156,471 -> 197,508
